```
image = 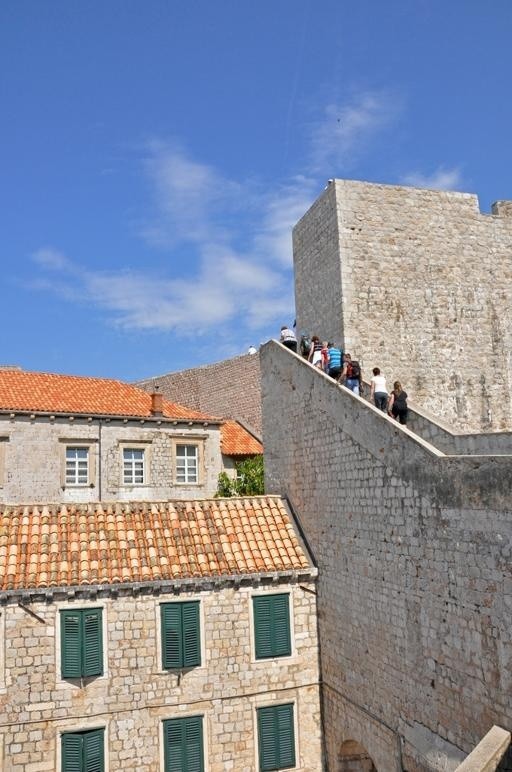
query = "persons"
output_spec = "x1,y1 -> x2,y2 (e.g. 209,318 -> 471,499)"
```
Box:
387,381 -> 409,428
369,368 -> 390,411
247,345 -> 257,355
281,325 -> 363,396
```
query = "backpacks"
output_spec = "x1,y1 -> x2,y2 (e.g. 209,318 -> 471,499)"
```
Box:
344,361 -> 360,379
